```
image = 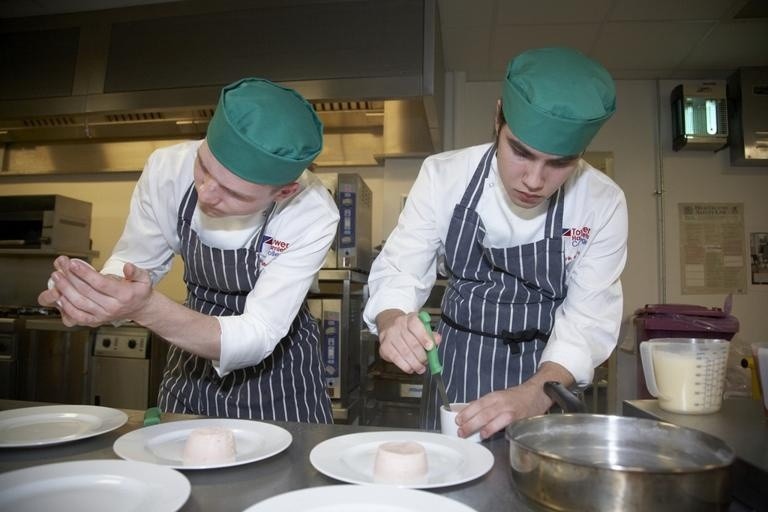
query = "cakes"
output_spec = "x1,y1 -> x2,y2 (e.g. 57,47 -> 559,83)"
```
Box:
374,443 -> 427,475
183,427 -> 237,462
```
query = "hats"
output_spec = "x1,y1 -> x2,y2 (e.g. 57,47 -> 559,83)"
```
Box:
501,47 -> 617,157
205,77 -> 324,187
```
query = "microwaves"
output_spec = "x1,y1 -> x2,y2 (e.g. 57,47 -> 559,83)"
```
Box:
0,194 -> 92,256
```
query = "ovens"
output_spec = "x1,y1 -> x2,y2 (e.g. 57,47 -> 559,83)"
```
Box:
299,172 -> 374,268
0,330 -> 21,401
296,295 -> 360,404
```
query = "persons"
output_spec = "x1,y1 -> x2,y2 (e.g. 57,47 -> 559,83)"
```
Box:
36,76 -> 340,425
363,47 -> 630,440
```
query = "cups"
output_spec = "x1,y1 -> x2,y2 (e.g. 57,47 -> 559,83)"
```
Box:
750,342 -> 768,412
638,340 -> 731,414
439,400 -> 485,444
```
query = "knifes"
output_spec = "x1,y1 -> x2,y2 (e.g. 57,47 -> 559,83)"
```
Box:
419,311 -> 451,412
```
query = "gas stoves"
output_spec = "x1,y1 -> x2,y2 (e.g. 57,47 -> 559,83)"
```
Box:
0,305 -> 61,324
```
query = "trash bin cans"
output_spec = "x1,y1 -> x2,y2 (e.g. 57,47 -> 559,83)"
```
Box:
634,304 -> 739,401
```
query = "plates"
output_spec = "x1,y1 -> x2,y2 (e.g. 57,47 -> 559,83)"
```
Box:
2,459 -> 190,512
0,405 -> 128,449
309,430 -> 494,486
242,483 -> 478,512
114,418 -> 293,471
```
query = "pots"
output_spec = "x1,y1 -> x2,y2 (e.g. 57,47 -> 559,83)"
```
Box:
505,379 -> 739,508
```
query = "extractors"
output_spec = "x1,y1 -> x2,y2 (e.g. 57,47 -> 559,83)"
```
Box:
24,96 -> 385,131
